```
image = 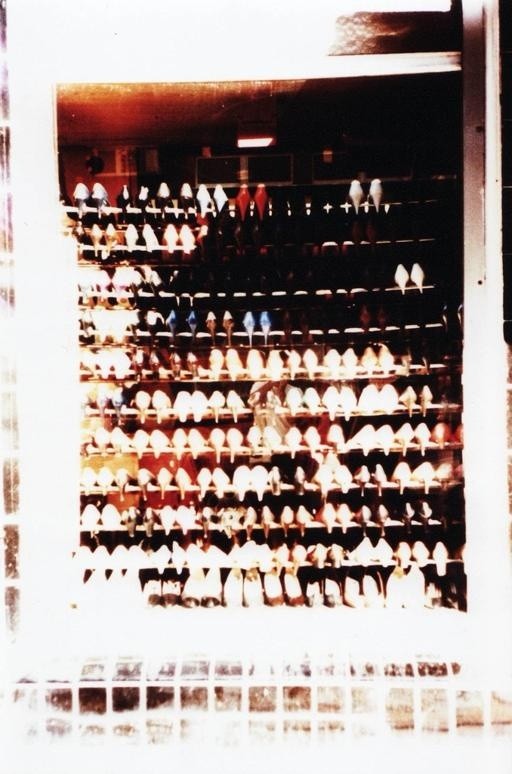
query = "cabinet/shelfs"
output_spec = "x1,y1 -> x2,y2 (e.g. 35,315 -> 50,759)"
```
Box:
58,199 -> 464,583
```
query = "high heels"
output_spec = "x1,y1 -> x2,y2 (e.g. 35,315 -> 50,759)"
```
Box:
61,179 -> 460,606
